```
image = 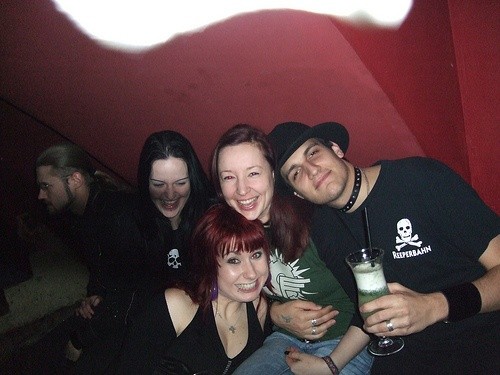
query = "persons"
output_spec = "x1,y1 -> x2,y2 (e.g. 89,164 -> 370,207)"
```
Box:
263,120 -> 500,375
108,198 -> 285,374
33,143 -> 162,375
49,129 -> 215,374
204,123 -> 381,375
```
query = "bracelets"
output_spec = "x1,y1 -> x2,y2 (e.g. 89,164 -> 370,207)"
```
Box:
321,355 -> 339,375
440,280 -> 482,326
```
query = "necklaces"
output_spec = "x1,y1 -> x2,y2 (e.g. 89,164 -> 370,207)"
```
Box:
211,295 -> 247,334
335,164 -> 362,215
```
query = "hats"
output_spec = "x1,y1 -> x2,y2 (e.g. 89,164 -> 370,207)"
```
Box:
265,121 -> 350,193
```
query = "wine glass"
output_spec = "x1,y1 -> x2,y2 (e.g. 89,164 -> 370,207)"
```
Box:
345,248 -> 404,355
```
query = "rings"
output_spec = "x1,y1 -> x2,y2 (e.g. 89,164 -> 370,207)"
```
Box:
312,328 -> 317,335
311,319 -> 316,327
385,319 -> 394,332
